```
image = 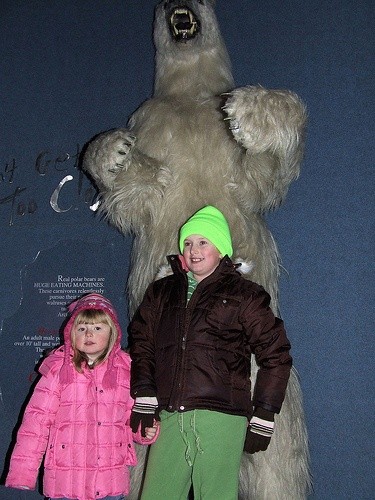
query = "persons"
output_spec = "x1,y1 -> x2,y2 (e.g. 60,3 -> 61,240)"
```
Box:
128,206 -> 293,500
6,293 -> 157,500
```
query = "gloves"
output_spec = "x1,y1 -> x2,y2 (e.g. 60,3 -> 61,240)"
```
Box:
243,410 -> 276,455
129,396 -> 160,436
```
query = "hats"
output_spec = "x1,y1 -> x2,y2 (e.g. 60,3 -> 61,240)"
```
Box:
57,293 -> 122,390
177,206 -> 235,258
